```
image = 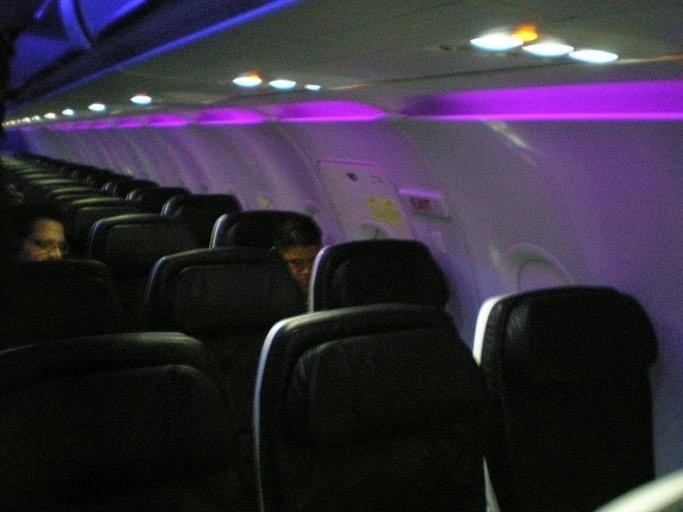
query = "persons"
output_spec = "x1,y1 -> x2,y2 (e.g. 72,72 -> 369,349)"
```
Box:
0,205 -> 66,261
276,222 -> 322,293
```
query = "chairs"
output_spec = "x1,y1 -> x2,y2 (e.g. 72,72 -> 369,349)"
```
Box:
0,154 -> 659,512
